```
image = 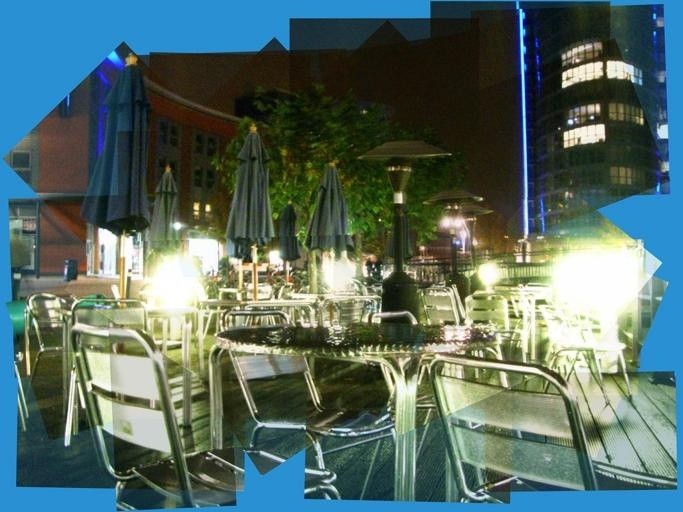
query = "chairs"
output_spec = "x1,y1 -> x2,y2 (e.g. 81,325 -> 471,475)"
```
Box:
64,298 -> 150,446
20,292 -> 71,396
426,352 -> 678,501
172,282 -> 631,403
218,309 -> 400,500
70,319 -> 345,512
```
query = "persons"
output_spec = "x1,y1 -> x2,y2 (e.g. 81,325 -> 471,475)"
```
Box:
80,53 -> 354,302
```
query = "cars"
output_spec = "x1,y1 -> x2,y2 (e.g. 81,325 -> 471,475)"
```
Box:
243,266 -> 273,300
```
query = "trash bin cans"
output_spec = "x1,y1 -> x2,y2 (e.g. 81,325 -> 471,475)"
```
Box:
64,259 -> 77,281
